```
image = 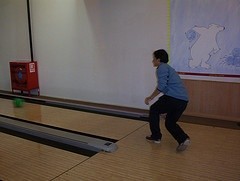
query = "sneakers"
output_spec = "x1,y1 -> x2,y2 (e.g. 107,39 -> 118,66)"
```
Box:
176,137 -> 191,152
145,136 -> 161,143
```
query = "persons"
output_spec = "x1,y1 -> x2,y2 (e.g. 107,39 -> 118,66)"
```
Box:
145,49 -> 191,151
